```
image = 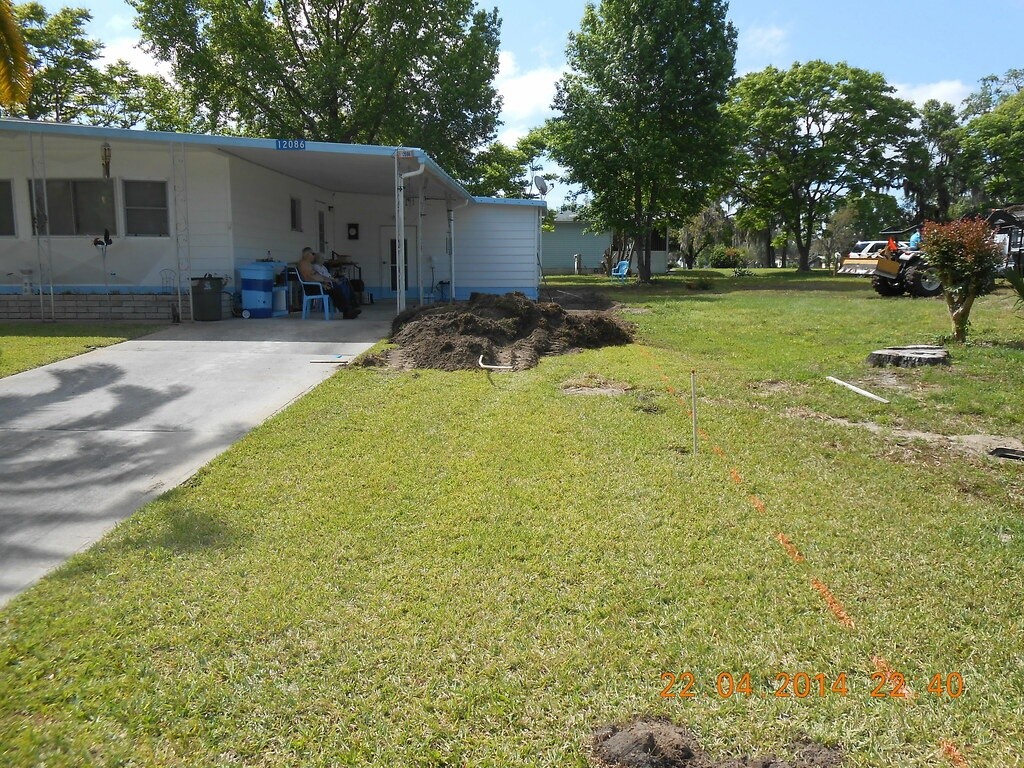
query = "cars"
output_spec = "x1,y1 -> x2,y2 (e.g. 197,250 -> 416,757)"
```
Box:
849,239 -> 911,258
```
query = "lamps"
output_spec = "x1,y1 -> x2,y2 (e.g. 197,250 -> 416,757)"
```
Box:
101,142 -> 112,182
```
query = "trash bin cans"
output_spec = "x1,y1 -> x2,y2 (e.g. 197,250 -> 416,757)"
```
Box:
237,262 -> 275,319
188,273 -> 224,322
573,253 -> 582,275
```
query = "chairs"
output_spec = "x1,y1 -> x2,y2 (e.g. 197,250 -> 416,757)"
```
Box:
295,266 -> 335,320
611,261 -> 630,284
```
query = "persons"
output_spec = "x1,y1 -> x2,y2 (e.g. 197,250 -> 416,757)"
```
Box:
910,229 -> 921,247
298,247 -> 361,319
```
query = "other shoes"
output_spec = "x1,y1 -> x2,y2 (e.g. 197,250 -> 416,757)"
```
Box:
343,308 -> 361,319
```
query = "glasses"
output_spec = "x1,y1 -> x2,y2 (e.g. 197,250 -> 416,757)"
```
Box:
310,252 -> 316,256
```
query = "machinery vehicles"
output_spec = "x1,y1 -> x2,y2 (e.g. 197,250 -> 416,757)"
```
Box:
872,238 -> 996,297
